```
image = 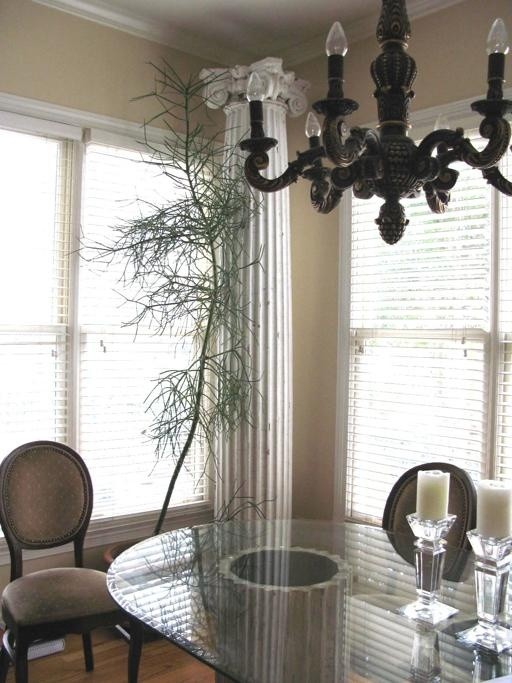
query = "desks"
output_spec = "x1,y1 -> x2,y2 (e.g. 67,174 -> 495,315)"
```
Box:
104,517 -> 511,683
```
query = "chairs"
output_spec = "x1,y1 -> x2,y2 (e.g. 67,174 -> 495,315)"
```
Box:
350,463 -> 476,683
0,441 -> 145,683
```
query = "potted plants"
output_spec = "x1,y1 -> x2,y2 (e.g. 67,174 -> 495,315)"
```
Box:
102,57 -> 273,640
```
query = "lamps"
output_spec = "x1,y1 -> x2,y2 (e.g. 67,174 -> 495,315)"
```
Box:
240,0 -> 512,245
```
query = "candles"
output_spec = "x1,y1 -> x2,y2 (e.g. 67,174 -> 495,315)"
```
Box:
476,478 -> 511,540
416,469 -> 449,520
